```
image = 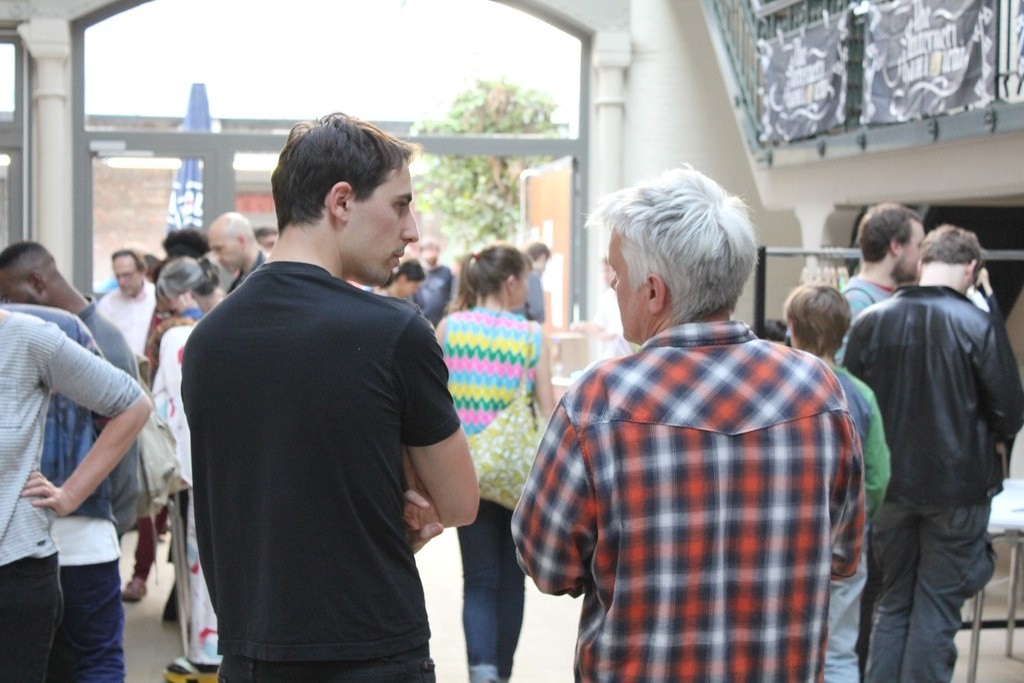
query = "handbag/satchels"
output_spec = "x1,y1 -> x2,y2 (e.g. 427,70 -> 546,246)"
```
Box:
134,350 -> 177,518
468,398 -> 541,511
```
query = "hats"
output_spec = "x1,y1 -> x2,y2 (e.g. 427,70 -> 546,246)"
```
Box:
163,227 -> 209,253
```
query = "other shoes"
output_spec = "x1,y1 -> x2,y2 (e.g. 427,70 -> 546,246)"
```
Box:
122,576 -> 147,602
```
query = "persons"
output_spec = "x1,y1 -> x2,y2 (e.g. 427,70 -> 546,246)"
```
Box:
121,230 -> 223,682
434,245 -> 568,683
373,260 -> 425,298
832,203 -> 927,370
785,281 -> 891,683
208,212 -> 269,294
0,309 -> 153,683
514,242 -> 550,323
414,238 -> 454,329
0,303 -> 153,682
254,227 -> 278,252
842,224 -> 1024,683
97,248 -> 157,358
0,240 -> 144,541
509,171 -> 866,683
183,112 -> 480,683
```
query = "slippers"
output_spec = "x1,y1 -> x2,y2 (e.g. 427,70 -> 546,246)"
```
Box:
164,656 -> 219,683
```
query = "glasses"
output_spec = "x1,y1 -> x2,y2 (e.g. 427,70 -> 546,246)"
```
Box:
169,308 -> 178,315
